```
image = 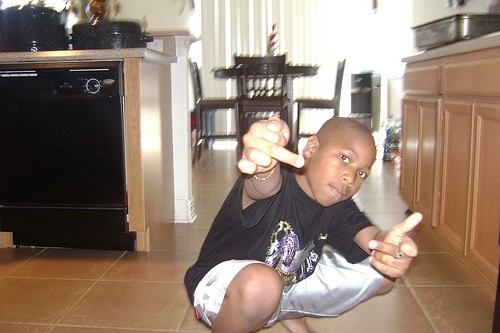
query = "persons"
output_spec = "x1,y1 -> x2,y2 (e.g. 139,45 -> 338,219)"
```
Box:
184,115 -> 423,333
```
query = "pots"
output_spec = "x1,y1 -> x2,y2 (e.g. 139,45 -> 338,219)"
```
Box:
0,4 -> 70,52
71,21 -> 153,49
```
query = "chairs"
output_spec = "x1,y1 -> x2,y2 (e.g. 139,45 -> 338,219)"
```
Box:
189,59 -> 235,163
234,52 -> 288,161
296,59 -> 349,148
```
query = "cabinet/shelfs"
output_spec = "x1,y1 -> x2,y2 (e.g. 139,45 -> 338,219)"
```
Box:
398,44 -> 500,305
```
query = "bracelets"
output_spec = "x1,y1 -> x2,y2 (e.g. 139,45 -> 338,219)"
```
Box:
254,168 -> 274,181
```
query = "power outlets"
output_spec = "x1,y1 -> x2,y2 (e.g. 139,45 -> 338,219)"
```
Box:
146,39 -> 163,53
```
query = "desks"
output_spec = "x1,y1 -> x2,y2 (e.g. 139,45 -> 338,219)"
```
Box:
213,64 -> 319,153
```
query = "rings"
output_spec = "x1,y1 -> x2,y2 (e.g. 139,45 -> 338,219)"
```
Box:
394,251 -> 403,259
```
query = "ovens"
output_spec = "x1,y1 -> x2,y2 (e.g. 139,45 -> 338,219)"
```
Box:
0,58 -> 137,250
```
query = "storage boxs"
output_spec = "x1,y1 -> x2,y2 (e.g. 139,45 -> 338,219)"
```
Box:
411,13 -> 500,50
